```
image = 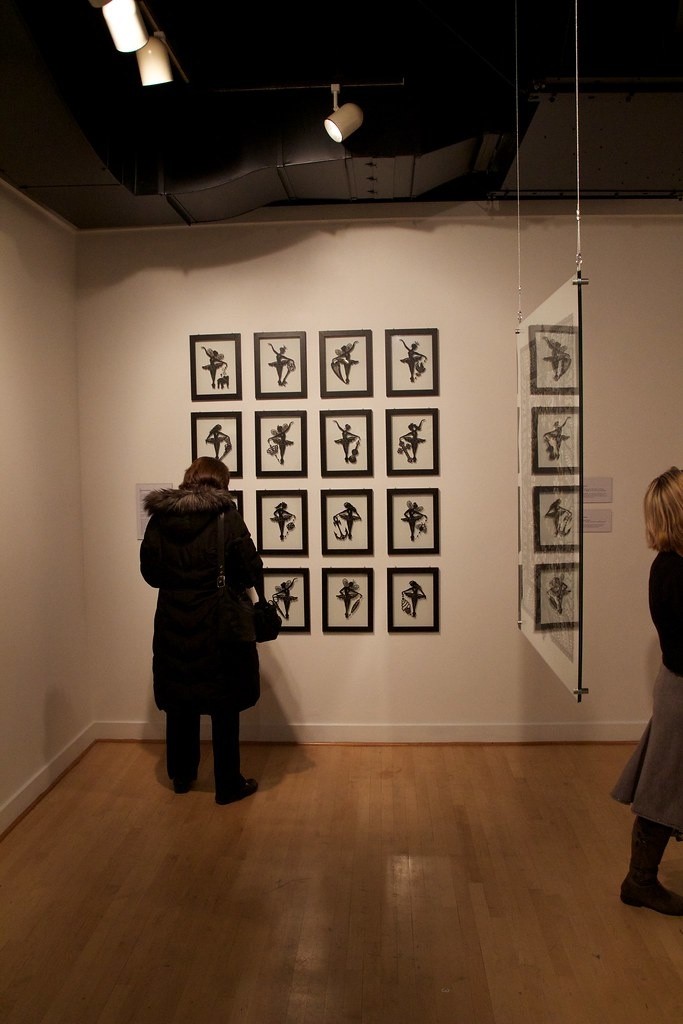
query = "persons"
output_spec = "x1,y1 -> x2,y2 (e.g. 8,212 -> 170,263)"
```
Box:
140,457 -> 282,803
609,467 -> 683,916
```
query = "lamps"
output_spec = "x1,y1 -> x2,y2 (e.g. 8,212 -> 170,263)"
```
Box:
324,84 -> 364,143
89,0 -> 174,87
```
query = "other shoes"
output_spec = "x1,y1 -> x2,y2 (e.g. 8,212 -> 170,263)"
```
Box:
215,778 -> 258,805
174,769 -> 198,794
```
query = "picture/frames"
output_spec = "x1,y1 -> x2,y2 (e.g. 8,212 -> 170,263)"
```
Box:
191,328 -> 441,633
530,322 -> 575,635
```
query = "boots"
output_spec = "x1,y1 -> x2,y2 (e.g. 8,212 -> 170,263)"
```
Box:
620,816 -> 683,917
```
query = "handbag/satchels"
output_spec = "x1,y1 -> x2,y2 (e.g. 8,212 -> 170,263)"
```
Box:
195,512 -> 282,643
